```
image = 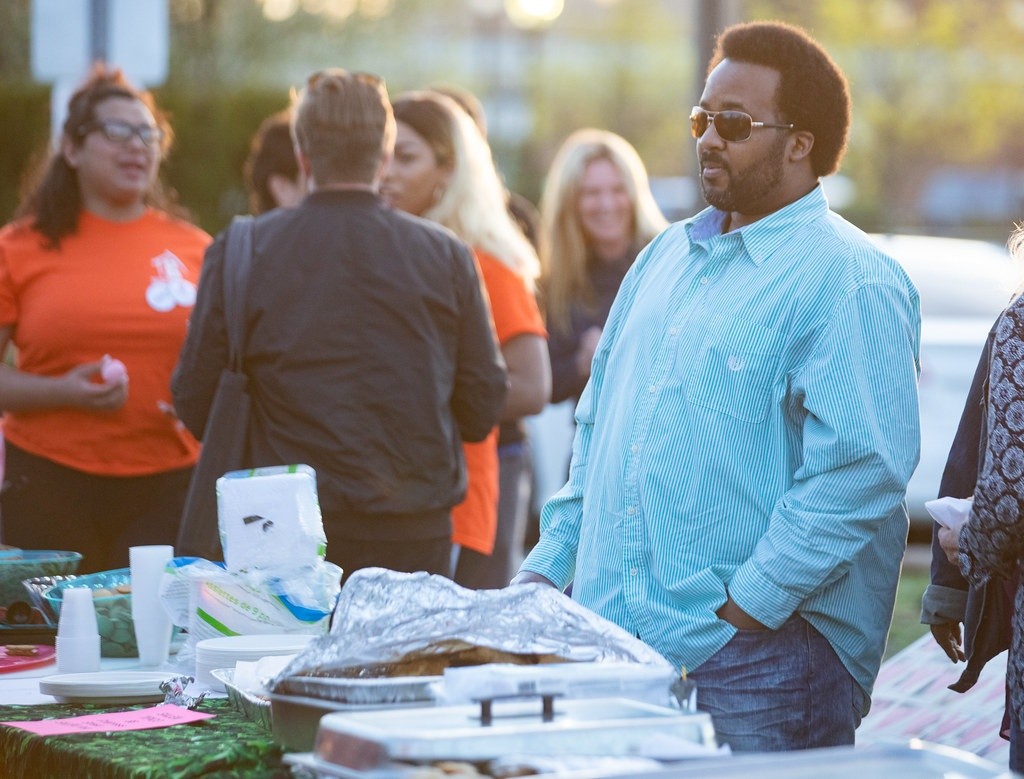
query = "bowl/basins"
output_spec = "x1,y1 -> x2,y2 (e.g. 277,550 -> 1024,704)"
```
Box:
0,549 -> 144,658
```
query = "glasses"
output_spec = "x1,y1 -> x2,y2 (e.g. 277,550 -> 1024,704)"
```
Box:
687,106 -> 795,143
78,118 -> 161,146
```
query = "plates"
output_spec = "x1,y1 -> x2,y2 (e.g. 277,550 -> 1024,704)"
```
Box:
38,635 -> 317,705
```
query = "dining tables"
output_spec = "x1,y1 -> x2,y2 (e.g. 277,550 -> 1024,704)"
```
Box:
0,698 -> 297,779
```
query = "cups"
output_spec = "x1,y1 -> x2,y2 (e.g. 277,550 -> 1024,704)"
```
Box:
54,544 -> 174,675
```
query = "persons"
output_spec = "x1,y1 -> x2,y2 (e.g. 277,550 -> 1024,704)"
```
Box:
168,71 -> 512,581
921,219 -> 1024,777
504,24 -> 923,753
247,89 -> 667,590
0,61 -> 216,574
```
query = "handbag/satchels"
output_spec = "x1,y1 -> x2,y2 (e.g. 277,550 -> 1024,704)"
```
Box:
174,213 -> 255,561
